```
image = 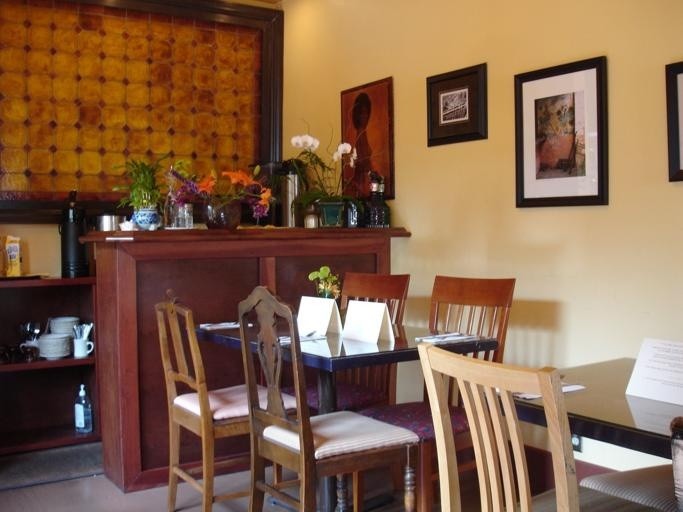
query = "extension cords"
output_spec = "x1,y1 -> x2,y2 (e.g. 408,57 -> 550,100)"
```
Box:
281,157 -> 313,229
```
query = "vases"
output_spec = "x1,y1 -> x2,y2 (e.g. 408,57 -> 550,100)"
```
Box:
307,266 -> 340,300
164,158 -> 292,219
272,116 -> 371,227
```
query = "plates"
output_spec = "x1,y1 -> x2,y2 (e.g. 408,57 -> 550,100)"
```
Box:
0,189 -> 234,458
78,223 -> 411,494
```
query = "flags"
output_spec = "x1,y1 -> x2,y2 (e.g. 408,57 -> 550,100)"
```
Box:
321,289 -> 332,299
201,198 -> 242,229
309,201 -> 346,227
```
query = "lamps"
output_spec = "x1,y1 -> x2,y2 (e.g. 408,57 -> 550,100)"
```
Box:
154,297 -> 316,512
280,271 -> 410,417
578,464 -> 681,512
352,275 -> 516,512
415,342 -> 666,512
237,285 -> 419,512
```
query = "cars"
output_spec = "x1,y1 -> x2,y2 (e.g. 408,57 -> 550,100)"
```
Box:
20,341 -> 41,363
166,204 -> 196,228
72,339 -> 94,360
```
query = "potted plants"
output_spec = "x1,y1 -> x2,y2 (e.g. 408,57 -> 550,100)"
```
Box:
513,55 -> 609,208
664,60 -> 682,182
340,76 -> 394,204
425,61 -> 489,148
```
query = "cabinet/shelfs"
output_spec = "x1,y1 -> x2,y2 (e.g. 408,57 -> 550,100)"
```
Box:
377,175 -> 390,227
365,173 -> 381,227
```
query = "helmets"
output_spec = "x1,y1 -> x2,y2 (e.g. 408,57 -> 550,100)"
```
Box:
38,333 -> 72,361
49,316 -> 81,340
164,226 -> 198,231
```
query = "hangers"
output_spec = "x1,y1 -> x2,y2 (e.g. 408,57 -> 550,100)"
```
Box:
17,321 -> 42,343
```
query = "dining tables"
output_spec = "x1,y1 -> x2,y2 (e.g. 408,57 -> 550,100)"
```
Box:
110,150 -> 173,229
